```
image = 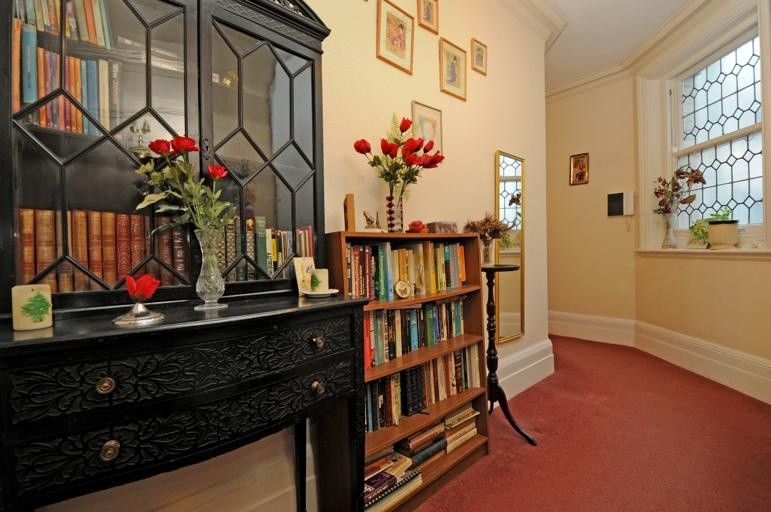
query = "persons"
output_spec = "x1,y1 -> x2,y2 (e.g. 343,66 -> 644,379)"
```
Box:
447,55 -> 458,83
392,23 -> 404,50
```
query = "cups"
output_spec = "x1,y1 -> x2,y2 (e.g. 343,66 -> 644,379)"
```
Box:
13,284 -> 53,330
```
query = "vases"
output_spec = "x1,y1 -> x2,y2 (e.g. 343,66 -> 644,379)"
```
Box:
481,234 -> 496,267
192,228 -> 232,313
386,190 -> 405,233
660,211 -> 679,249
132,300 -> 151,318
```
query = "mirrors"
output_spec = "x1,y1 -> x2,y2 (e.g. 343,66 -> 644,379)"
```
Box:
493,148 -> 527,345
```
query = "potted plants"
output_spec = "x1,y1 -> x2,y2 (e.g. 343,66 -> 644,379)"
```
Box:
688,206 -> 742,250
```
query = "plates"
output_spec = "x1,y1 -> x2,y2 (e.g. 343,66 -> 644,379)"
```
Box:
406,228 -> 430,233
395,280 -> 412,298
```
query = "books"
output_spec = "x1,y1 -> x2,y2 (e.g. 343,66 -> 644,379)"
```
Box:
20,207 -> 316,293
343,241 -> 483,512
12,0 -> 123,137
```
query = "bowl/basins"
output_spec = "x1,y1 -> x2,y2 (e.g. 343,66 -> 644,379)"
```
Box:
304,289 -> 340,297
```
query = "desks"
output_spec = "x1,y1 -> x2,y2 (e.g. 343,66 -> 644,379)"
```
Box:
481,263 -> 540,447
1,293 -> 371,510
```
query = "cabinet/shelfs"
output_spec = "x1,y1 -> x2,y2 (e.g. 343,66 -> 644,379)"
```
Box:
323,230 -> 493,511
0,1 -> 333,328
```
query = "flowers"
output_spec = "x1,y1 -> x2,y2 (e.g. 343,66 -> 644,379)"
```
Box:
351,110 -> 447,226
125,272 -> 162,302
463,209 -> 518,242
129,132 -> 238,296
651,165 -> 708,214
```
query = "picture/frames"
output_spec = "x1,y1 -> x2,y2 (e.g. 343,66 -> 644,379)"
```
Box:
376,0 -> 417,75
411,100 -> 444,157
439,35 -> 469,103
471,37 -> 490,76
417,0 -> 441,36
569,151 -> 590,186
